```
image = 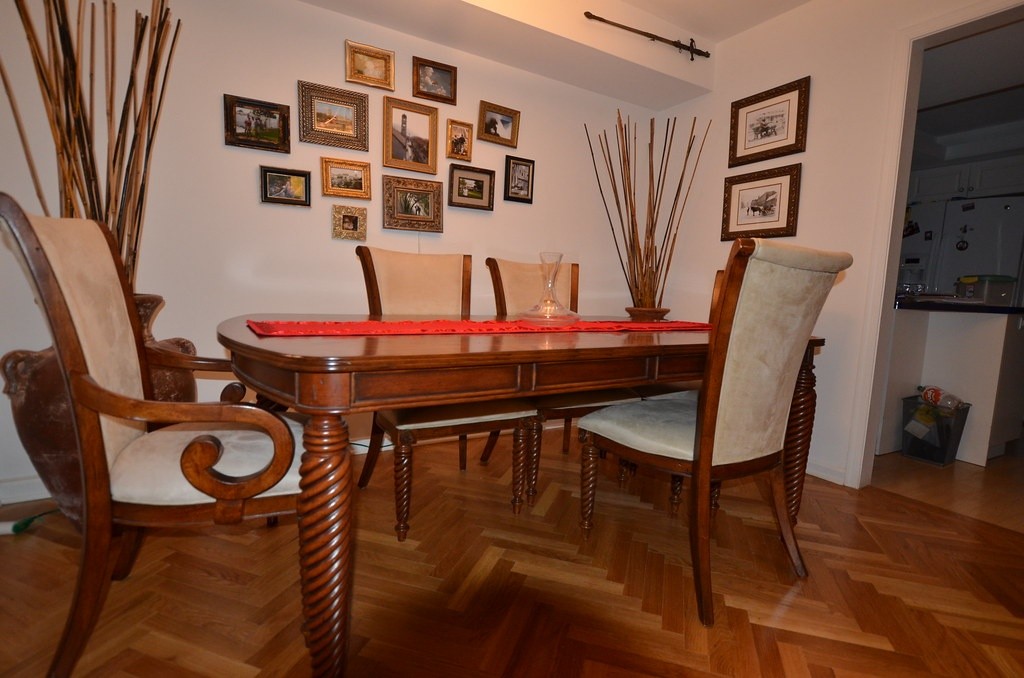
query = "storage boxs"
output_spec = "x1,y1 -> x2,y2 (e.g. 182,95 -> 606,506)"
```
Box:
954,274 -> 1018,306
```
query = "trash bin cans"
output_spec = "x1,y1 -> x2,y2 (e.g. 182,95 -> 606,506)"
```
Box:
901,395 -> 972,466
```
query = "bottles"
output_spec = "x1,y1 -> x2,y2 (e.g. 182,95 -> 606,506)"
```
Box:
917,385 -> 961,409
516,252 -> 582,322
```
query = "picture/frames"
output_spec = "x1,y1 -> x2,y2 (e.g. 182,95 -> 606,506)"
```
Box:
721,76 -> 812,242
224,39 -> 534,242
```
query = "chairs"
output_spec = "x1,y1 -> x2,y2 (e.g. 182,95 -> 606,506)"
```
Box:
0,189 -> 855,678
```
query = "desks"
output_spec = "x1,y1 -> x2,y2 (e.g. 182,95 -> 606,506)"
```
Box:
218,313 -> 827,678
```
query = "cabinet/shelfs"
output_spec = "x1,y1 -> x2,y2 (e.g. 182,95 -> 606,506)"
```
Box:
906,153 -> 1024,204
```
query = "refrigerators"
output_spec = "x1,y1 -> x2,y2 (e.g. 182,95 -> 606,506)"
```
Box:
896,196 -> 1024,308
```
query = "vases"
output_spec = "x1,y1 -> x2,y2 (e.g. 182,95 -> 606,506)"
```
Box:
625,307 -> 671,321
0,293 -> 196,536
